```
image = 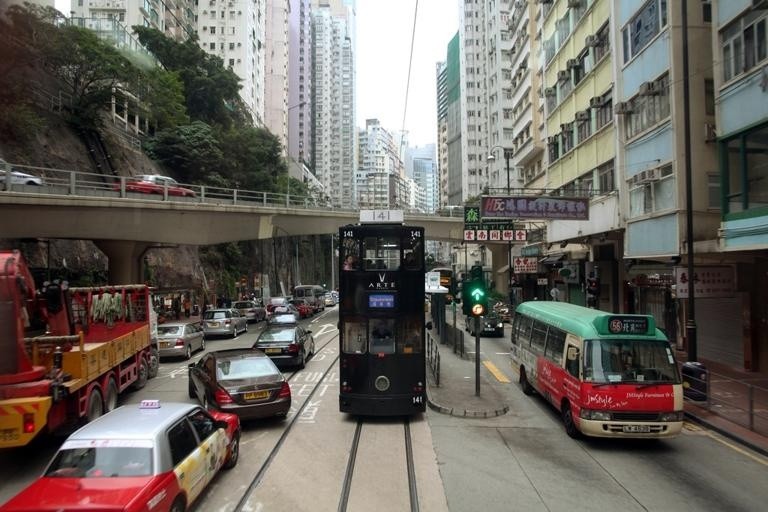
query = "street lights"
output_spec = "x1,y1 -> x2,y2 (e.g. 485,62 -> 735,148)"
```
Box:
487,145 -> 510,196
286,101 -> 307,194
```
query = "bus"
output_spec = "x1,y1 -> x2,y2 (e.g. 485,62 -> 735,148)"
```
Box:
430,267 -> 453,291
337,223 -> 427,415
510,301 -> 690,440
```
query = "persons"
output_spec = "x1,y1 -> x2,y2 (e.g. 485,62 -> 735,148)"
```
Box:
342,255 -> 353,270
216,295 -> 232,308
183,298 -> 191,318
202,304 -> 207,320
174,298 -> 181,319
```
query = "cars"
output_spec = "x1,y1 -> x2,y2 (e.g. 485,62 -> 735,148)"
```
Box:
465,313 -> 505,337
0,169 -> 42,185
113,174 -> 196,197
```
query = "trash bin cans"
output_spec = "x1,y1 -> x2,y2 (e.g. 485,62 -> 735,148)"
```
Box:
681,361 -> 708,406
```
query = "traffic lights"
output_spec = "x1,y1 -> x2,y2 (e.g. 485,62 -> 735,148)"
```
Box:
585,277 -> 600,307
463,265 -> 488,315
